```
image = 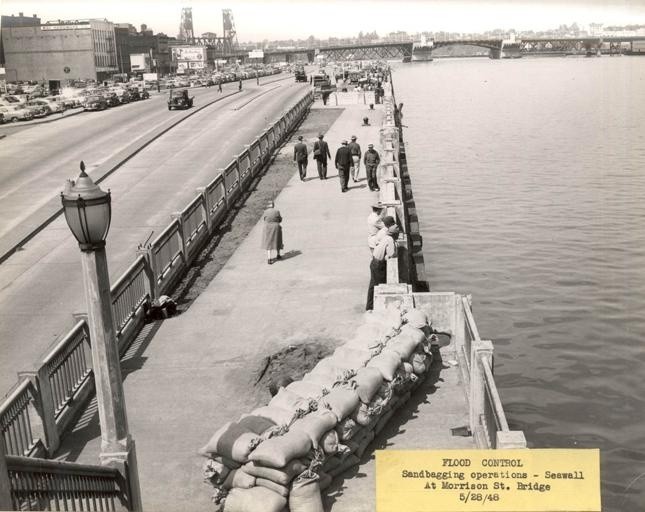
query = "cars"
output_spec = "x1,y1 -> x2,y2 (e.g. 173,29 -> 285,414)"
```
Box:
0,81 -> 85,122
84,76 -> 148,111
166,62 -> 291,87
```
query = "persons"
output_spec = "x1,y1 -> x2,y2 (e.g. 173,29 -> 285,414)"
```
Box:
322,93 -> 327,104
313,134 -> 331,180
348,136 -> 361,182
238,78 -> 242,90
294,136 -> 308,182
335,141 -> 354,192
366,200 -> 404,311
216,80 -> 221,93
262,201 -> 284,264
394,103 -> 404,128
364,144 -> 380,192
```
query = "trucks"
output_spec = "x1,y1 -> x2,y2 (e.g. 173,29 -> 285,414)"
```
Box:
168,89 -> 195,110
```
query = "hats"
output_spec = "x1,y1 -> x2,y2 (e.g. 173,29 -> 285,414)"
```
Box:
341,140 -> 347,144
368,144 -> 373,148
370,201 -> 385,209
387,224 -> 400,235
351,136 -> 356,140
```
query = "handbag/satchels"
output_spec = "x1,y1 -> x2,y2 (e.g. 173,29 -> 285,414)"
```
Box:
314,149 -> 322,156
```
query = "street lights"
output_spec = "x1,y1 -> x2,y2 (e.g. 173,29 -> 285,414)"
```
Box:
58,162 -> 144,507
